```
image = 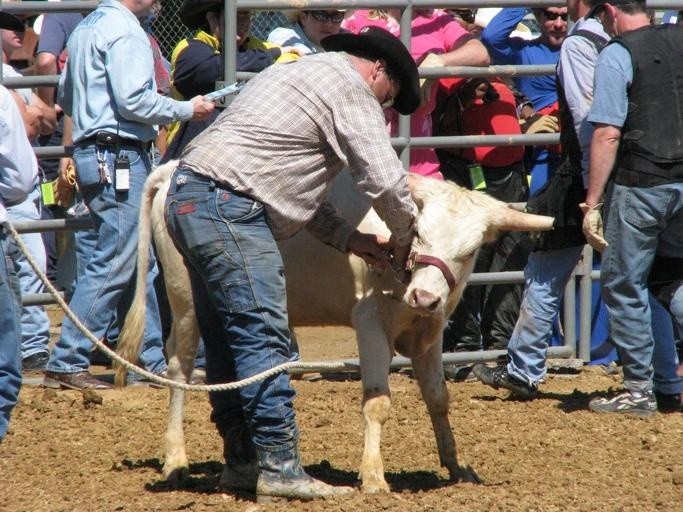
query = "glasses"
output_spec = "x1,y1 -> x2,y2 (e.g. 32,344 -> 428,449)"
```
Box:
544,11 -> 567,21
311,11 -> 344,24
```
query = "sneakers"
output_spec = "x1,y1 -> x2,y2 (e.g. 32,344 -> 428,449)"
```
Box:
472,363 -> 537,400
589,390 -> 683,415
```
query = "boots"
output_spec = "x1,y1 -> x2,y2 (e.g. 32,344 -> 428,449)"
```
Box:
219,440 -> 355,504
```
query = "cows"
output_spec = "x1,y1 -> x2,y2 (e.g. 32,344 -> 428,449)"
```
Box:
110,157 -> 558,495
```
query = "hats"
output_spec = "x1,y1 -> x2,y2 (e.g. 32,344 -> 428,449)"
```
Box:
320,26 -> 420,115
180,0 -> 225,34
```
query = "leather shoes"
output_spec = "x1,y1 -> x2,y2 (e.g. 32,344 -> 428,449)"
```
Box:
43,371 -> 115,391
21,352 -> 49,373
126,368 -> 206,387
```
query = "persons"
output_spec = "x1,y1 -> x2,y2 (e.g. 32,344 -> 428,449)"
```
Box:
165,26 -> 421,497
45,0 -> 215,390
0,84 -> 40,440
432,7 -> 682,366
579,0 -> 683,413
268,9 -> 347,54
473,0 -> 683,397
341,7 -> 490,180
173,0 -> 304,386
0,0 -> 173,368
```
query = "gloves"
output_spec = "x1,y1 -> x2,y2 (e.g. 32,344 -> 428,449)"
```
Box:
579,203 -> 609,253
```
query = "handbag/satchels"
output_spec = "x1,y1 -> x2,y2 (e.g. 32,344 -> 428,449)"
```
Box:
520,161 -> 587,251
